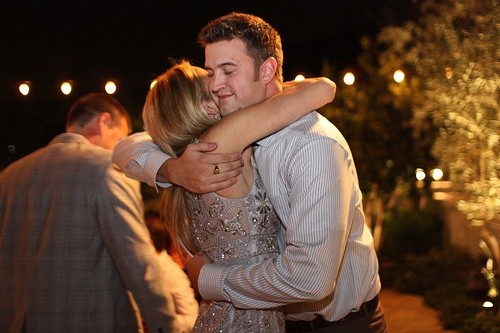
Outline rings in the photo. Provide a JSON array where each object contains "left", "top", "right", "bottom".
[{"left": 214, "top": 165, "right": 221, "bottom": 174}]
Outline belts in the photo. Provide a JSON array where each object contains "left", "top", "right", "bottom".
[{"left": 285, "top": 295, "right": 378, "bottom": 333}]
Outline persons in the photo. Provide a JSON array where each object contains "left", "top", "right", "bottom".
[
  {"left": 0, "top": 62, "right": 337, "bottom": 333},
  {"left": 112, "top": 12, "right": 388, "bottom": 333}
]
